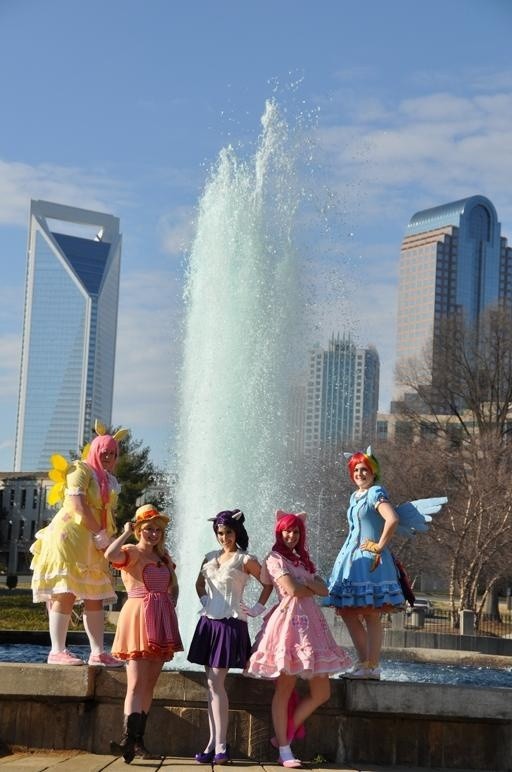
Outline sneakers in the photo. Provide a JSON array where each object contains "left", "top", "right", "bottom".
[
  {"left": 87, "top": 651, "right": 125, "bottom": 667},
  {"left": 339, "top": 663, "right": 381, "bottom": 680},
  {"left": 270, "top": 736, "right": 301, "bottom": 767},
  {"left": 48, "top": 648, "right": 83, "bottom": 665}
]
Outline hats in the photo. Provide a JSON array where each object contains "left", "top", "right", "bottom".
[{"left": 131, "top": 504, "right": 170, "bottom": 529}]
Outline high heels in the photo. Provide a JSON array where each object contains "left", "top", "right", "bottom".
[
  {"left": 195, "top": 746, "right": 214, "bottom": 763},
  {"left": 213, "top": 741, "right": 232, "bottom": 765}
]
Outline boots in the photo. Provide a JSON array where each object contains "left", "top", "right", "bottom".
[
  {"left": 109, "top": 711, "right": 141, "bottom": 764},
  {"left": 135, "top": 710, "right": 161, "bottom": 759}
]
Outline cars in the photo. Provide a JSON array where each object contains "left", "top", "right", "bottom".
[{"left": 406, "top": 598, "right": 436, "bottom": 618}]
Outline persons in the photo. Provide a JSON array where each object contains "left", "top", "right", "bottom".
[
  {"left": 245, "top": 515, "right": 353, "bottom": 768},
  {"left": 29, "top": 434, "right": 126, "bottom": 669},
  {"left": 104, "top": 502, "right": 184, "bottom": 762},
  {"left": 322, "top": 453, "right": 406, "bottom": 678},
  {"left": 187, "top": 509, "right": 273, "bottom": 762}
]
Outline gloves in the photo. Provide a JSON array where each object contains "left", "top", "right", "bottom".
[
  {"left": 197, "top": 596, "right": 210, "bottom": 615},
  {"left": 94, "top": 529, "right": 110, "bottom": 551},
  {"left": 240, "top": 600, "right": 266, "bottom": 617}
]
